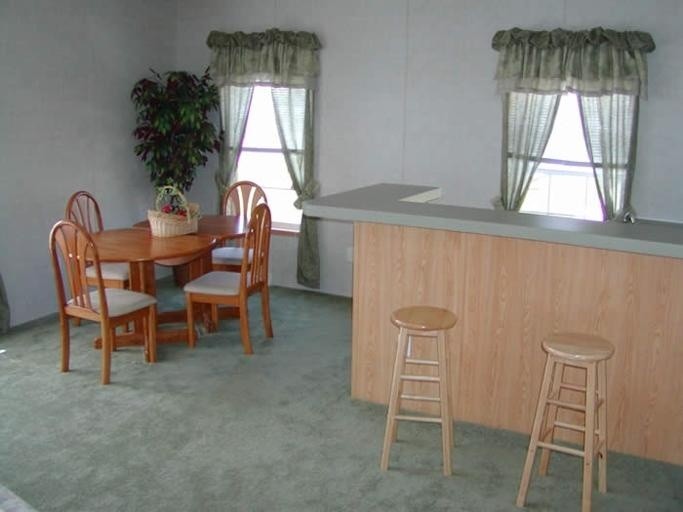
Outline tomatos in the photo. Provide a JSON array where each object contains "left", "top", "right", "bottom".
[{"left": 162, "top": 204, "right": 174, "bottom": 214}]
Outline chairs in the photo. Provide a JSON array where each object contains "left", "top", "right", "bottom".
[
  {"left": 181, "top": 203, "right": 274, "bottom": 356},
  {"left": 62, "top": 189, "right": 134, "bottom": 337},
  {"left": 48, "top": 218, "right": 159, "bottom": 387},
  {"left": 210, "top": 179, "right": 268, "bottom": 273}
]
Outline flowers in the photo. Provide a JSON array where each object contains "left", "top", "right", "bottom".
[
  {"left": 160, "top": 202, "right": 188, "bottom": 218},
  {"left": 128, "top": 61, "right": 225, "bottom": 287}
]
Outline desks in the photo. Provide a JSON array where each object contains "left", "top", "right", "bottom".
[{"left": 69, "top": 207, "right": 271, "bottom": 348}]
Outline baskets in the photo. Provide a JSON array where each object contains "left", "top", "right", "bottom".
[{"left": 147, "top": 186, "right": 200, "bottom": 238}]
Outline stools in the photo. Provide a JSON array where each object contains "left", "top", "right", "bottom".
[
  {"left": 375, "top": 304, "right": 460, "bottom": 479},
  {"left": 512, "top": 329, "right": 614, "bottom": 508}
]
